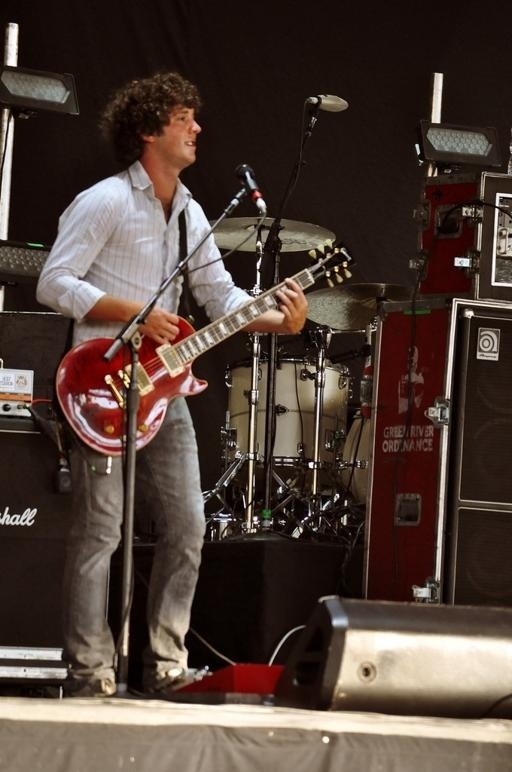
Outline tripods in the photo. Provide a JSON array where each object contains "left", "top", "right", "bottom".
[{"left": 200, "top": 229, "right": 366, "bottom": 543}]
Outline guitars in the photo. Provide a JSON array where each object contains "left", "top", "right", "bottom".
[{"left": 54, "top": 237, "right": 354, "bottom": 457}]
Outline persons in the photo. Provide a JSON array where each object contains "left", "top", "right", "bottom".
[{"left": 35, "top": 69, "right": 308, "bottom": 698}]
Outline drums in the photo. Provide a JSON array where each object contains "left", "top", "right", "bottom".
[
  {"left": 217, "top": 356, "right": 348, "bottom": 468},
  {"left": 335, "top": 412, "right": 374, "bottom": 506}
]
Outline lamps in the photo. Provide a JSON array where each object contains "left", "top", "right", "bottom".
[
  {"left": 0, "top": 66, "right": 80, "bottom": 129},
  {"left": 415, "top": 119, "right": 502, "bottom": 168}
]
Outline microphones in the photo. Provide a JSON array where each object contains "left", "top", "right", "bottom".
[
  {"left": 306, "top": 94, "right": 349, "bottom": 112},
  {"left": 236, "top": 163, "right": 267, "bottom": 212}
]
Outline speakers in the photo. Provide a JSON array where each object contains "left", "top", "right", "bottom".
[{"left": 361, "top": 298, "right": 512, "bottom": 607}]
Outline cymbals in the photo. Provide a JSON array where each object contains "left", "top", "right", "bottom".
[
  {"left": 206, "top": 217, "right": 338, "bottom": 254},
  {"left": 303, "top": 280, "right": 416, "bottom": 331}
]
